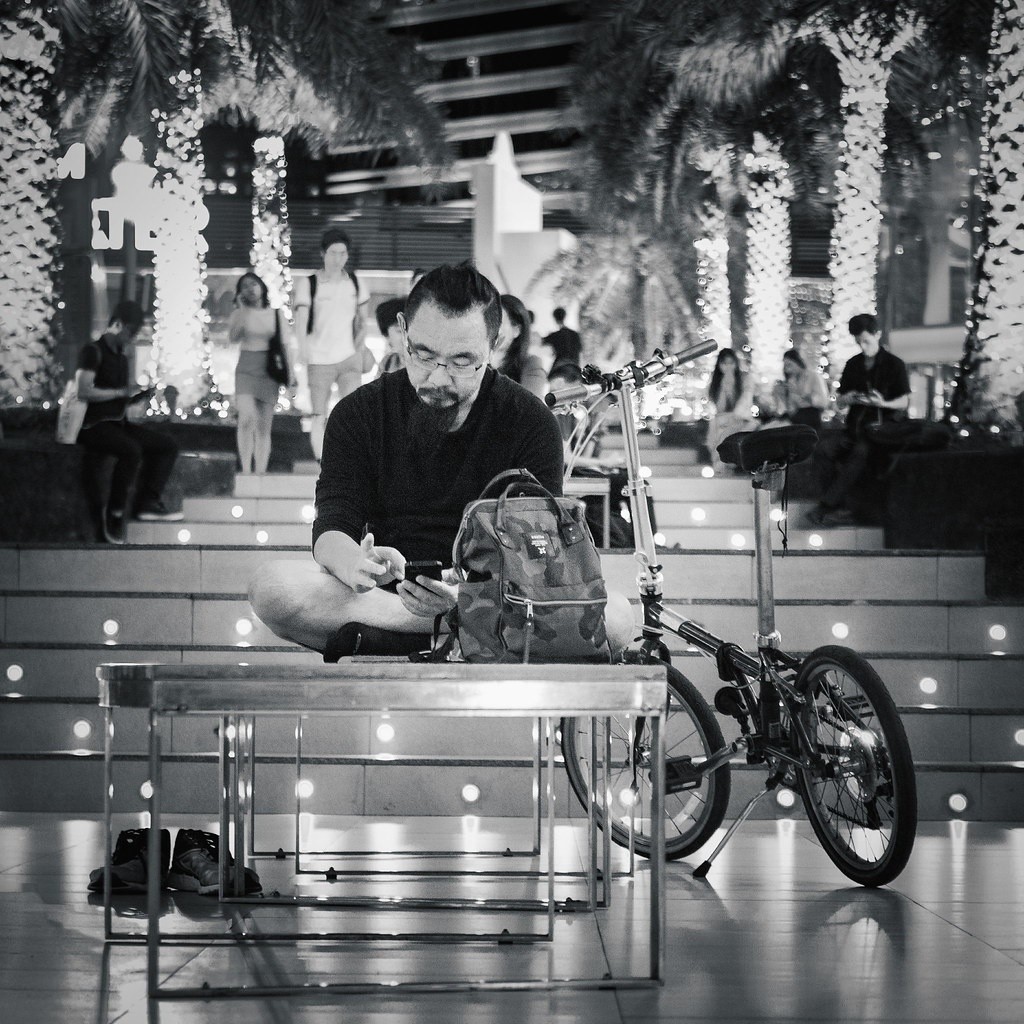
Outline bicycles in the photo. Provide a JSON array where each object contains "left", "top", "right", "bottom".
[{"left": 545, "top": 340, "right": 917, "bottom": 889}]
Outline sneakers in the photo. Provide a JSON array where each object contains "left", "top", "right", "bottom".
[
  {"left": 86, "top": 828, "right": 171, "bottom": 894},
  {"left": 168, "top": 828, "right": 262, "bottom": 896}
]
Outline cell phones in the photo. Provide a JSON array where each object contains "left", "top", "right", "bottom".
[{"left": 404, "top": 561, "right": 445, "bottom": 595}]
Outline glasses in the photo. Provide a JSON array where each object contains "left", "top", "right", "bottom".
[{"left": 404, "top": 327, "right": 489, "bottom": 378}]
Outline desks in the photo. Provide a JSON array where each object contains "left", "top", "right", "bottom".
[
  {"left": 564, "top": 476, "right": 610, "bottom": 549},
  {"left": 95, "top": 663, "right": 668, "bottom": 998}
]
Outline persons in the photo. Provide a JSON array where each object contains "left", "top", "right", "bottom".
[
  {"left": 228, "top": 272, "right": 296, "bottom": 475},
  {"left": 770, "top": 350, "right": 828, "bottom": 429},
  {"left": 296, "top": 228, "right": 372, "bottom": 466},
  {"left": 77, "top": 302, "right": 184, "bottom": 543},
  {"left": 246, "top": 257, "right": 664, "bottom": 656},
  {"left": 803, "top": 313, "right": 911, "bottom": 527},
  {"left": 706, "top": 349, "right": 760, "bottom": 470}
]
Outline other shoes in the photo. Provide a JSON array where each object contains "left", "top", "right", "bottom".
[
  {"left": 103, "top": 506, "right": 123, "bottom": 543},
  {"left": 804, "top": 508, "right": 830, "bottom": 524},
  {"left": 137, "top": 501, "right": 186, "bottom": 522}
]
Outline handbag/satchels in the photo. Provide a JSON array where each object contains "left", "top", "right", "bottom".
[
  {"left": 454, "top": 467, "right": 618, "bottom": 663},
  {"left": 56, "top": 345, "right": 101, "bottom": 443},
  {"left": 267, "top": 308, "right": 291, "bottom": 384}
]
